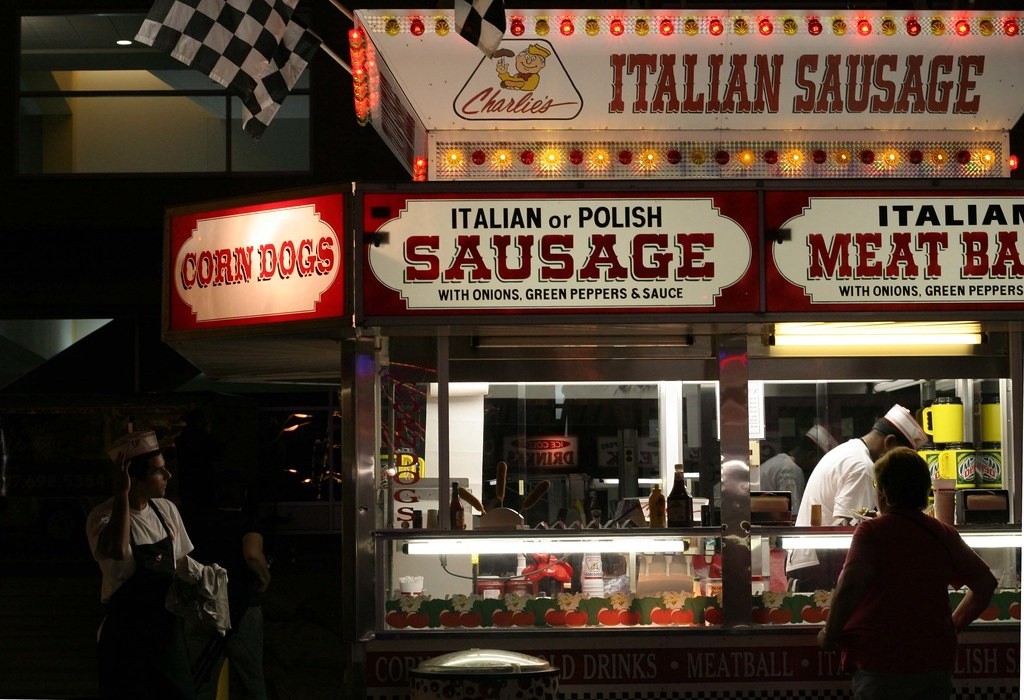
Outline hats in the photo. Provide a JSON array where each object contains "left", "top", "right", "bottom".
[
  {"left": 804, "top": 424, "right": 838, "bottom": 456},
  {"left": 107, "top": 431, "right": 158, "bottom": 464},
  {"left": 873, "top": 403, "right": 928, "bottom": 450}
]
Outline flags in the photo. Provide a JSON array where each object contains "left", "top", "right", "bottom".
[
  {"left": 454, "top": 1, "right": 508, "bottom": 59},
  {"left": 136, "top": 0, "right": 324, "bottom": 140}
]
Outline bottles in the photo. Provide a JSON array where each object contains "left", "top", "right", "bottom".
[
  {"left": 503, "top": 573, "right": 534, "bottom": 596},
  {"left": 919, "top": 441, "right": 975, "bottom": 489},
  {"left": 668, "top": 463, "right": 693, "bottom": 527},
  {"left": 475, "top": 575, "right": 504, "bottom": 599},
  {"left": 650, "top": 483, "right": 665, "bottom": 528},
  {"left": 449, "top": 481, "right": 463, "bottom": 532},
  {"left": 976, "top": 444, "right": 1002, "bottom": 488},
  {"left": 979, "top": 392, "right": 1001, "bottom": 441}
]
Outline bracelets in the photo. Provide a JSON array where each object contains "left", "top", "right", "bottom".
[{"left": 823, "top": 629, "right": 832, "bottom": 638}]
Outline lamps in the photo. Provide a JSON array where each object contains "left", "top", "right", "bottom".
[
  {"left": 469, "top": 336, "right": 697, "bottom": 349},
  {"left": 767, "top": 333, "right": 990, "bottom": 346}
]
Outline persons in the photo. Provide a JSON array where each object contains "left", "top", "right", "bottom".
[
  {"left": 793, "top": 403, "right": 929, "bottom": 528},
  {"left": 86, "top": 429, "right": 229, "bottom": 700},
  {"left": 760, "top": 424, "right": 839, "bottom": 523},
  {"left": 225, "top": 476, "right": 287, "bottom": 700},
  {"left": 815, "top": 447, "right": 998, "bottom": 700}
]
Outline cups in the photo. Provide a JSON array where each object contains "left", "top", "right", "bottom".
[
  {"left": 933, "top": 489, "right": 955, "bottom": 526},
  {"left": 921, "top": 396, "right": 965, "bottom": 444},
  {"left": 411, "top": 510, "right": 422, "bottom": 528},
  {"left": 427, "top": 509, "right": 438, "bottom": 528},
  {"left": 812, "top": 504, "right": 822, "bottom": 526}
]
